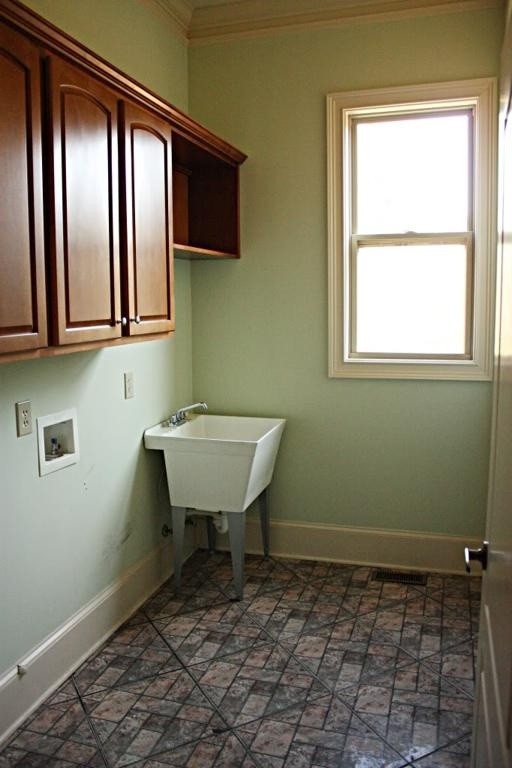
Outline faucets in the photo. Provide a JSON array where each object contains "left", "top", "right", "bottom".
[{"left": 176, "top": 401, "right": 209, "bottom": 420}]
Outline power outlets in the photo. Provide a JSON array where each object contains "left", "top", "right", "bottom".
[
  {"left": 15, "top": 399, "right": 32, "bottom": 436},
  {"left": 124, "top": 371, "right": 135, "bottom": 398}
]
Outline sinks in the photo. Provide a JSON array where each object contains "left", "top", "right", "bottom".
[{"left": 143, "top": 412, "right": 288, "bottom": 513}]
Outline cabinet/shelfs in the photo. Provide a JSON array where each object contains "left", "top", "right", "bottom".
[
  {"left": 0, "top": 21, "right": 49, "bottom": 353},
  {"left": 171, "top": 128, "right": 239, "bottom": 257},
  {"left": 48, "top": 59, "right": 173, "bottom": 346}
]
